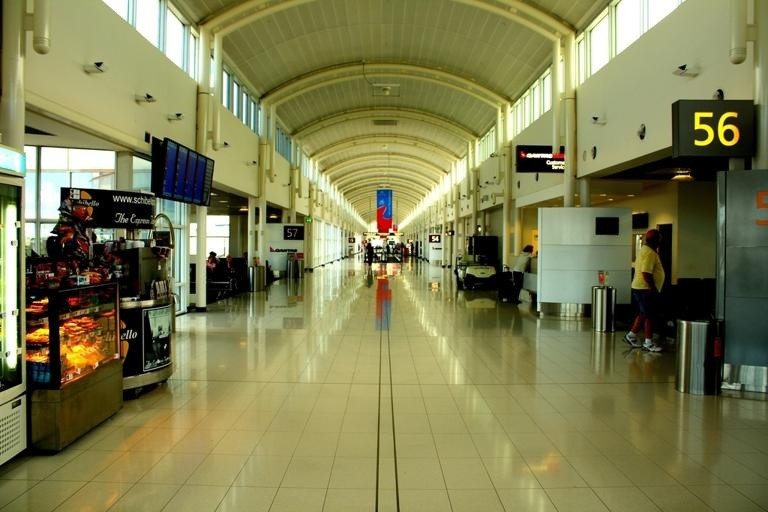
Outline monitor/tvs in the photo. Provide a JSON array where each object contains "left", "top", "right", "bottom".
[{"left": 632, "top": 213, "right": 648, "bottom": 229}]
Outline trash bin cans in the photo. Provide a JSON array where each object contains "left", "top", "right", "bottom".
[
  {"left": 592, "top": 286, "right": 617, "bottom": 332},
  {"left": 250, "top": 266, "right": 264, "bottom": 292},
  {"left": 675, "top": 320, "right": 725, "bottom": 395},
  {"left": 286, "top": 260, "right": 304, "bottom": 278}
]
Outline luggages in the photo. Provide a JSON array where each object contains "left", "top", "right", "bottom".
[{"left": 497, "top": 266, "right": 512, "bottom": 302}]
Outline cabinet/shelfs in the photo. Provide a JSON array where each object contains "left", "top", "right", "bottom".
[{"left": 26, "top": 283, "right": 123, "bottom": 457}]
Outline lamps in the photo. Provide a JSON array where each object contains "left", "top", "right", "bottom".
[
  {"left": 248, "top": 161, "right": 256, "bottom": 166},
  {"left": 674, "top": 62, "right": 699, "bottom": 74},
  {"left": 592, "top": 116, "right": 606, "bottom": 123},
  {"left": 363, "top": 61, "right": 400, "bottom": 97},
  {"left": 136, "top": 93, "right": 156, "bottom": 102},
  {"left": 220, "top": 141, "right": 230, "bottom": 148},
  {"left": 85, "top": 62, "right": 107, "bottom": 72},
  {"left": 168, "top": 113, "right": 184, "bottom": 120}
]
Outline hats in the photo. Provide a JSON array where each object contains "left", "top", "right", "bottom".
[{"left": 646, "top": 229, "right": 663, "bottom": 240}]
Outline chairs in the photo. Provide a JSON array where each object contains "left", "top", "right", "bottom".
[{"left": 190, "top": 258, "right": 249, "bottom": 304}]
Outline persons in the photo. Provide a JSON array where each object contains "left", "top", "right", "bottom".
[
  {"left": 386, "top": 244, "right": 390, "bottom": 254},
  {"left": 510, "top": 245, "right": 538, "bottom": 304},
  {"left": 207, "top": 252, "right": 219, "bottom": 272},
  {"left": 367, "top": 243, "right": 374, "bottom": 266},
  {"left": 623, "top": 230, "right": 669, "bottom": 352},
  {"left": 223, "top": 256, "right": 235, "bottom": 276}
]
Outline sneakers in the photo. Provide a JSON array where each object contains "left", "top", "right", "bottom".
[{"left": 623, "top": 334, "right": 664, "bottom": 352}]
